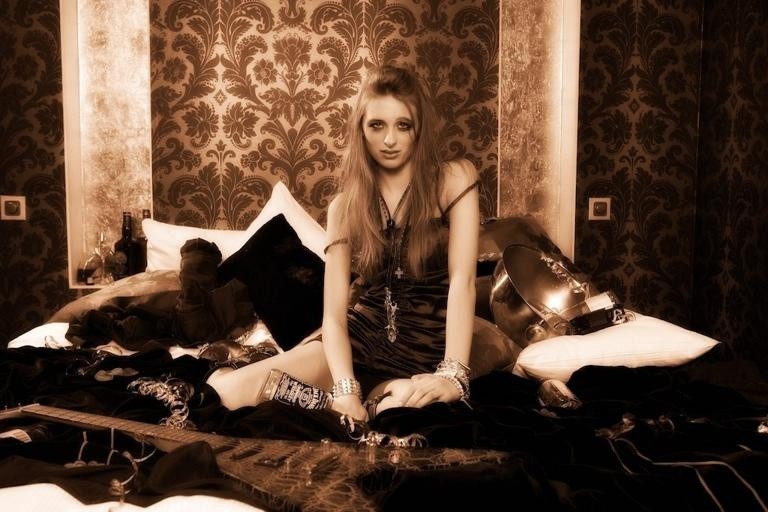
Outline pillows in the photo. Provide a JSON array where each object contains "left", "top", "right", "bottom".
[
  {"left": 518, "top": 316, "right": 719, "bottom": 384},
  {"left": 141, "top": 181, "right": 356, "bottom": 350}
]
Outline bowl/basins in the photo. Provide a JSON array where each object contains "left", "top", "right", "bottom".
[{"left": 488, "top": 242, "right": 592, "bottom": 349}]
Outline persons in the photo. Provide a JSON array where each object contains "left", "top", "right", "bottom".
[{"left": 194, "top": 65, "right": 482, "bottom": 423}]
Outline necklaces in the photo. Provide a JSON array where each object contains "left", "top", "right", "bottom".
[{"left": 372, "top": 180, "right": 415, "bottom": 342}]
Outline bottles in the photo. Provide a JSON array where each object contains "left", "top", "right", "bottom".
[
  {"left": 114, "top": 207, "right": 153, "bottom": 280},
  {"left": 77, "top": 231, "right": 115, "bottom": 285},
  {"left": 535, "top": 376, "right": 584, "bottom": 411},
  {"left": 256, "top": 368, "right": 334, "bottom": 410}
]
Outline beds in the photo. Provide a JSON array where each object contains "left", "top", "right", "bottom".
[{"left": 0, "top": 270, "right": 768, "bottom": 512}]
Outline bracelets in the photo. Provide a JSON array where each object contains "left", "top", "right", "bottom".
[
  {"left": 433, "top": 356, "right": 473, "bottom": 399},
  {"left": 331, "top": 378, "right": 364, "bottom": 401}
]
